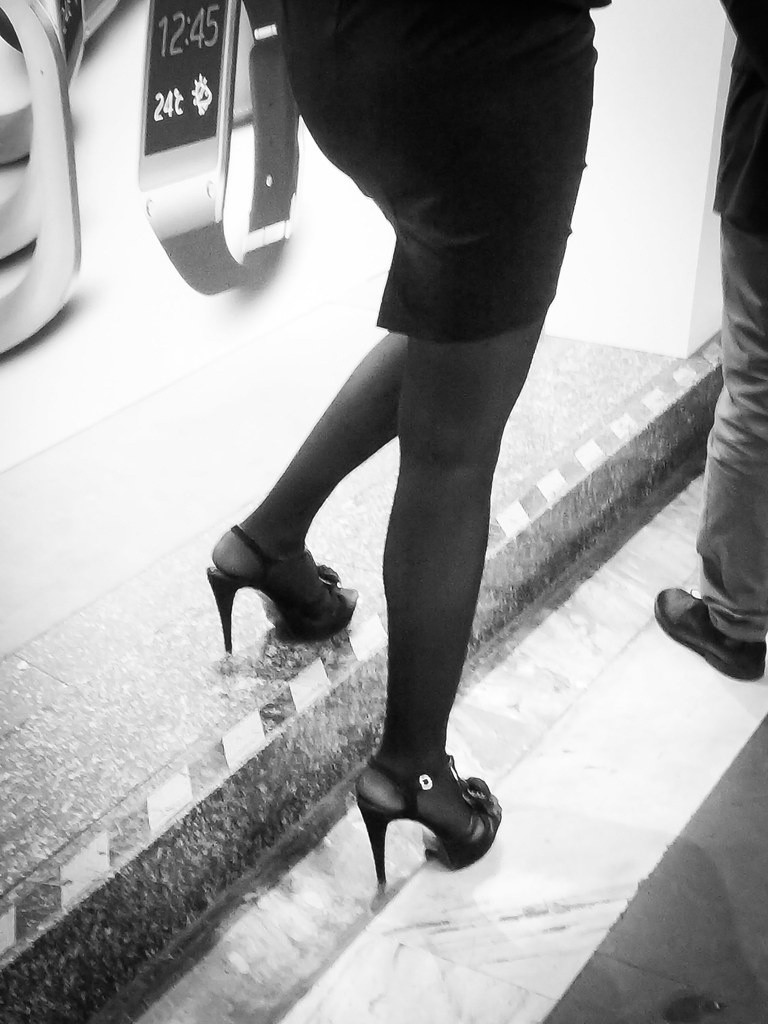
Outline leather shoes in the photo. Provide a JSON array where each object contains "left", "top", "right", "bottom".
[{"left": 655, "top": 588, "right": 768, "bottom": 681}]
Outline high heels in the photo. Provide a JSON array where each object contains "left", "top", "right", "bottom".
[
  {"left": 356, "top": 753, "right": 503, "bottom": 883},
  {"left": 206, "top": 525, "right": 358, "bottom": 654}
]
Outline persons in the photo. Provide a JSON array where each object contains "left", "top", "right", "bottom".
[
  {"left": 204, "top": 0, "right": 613, "bottom": 886},
  {"left": 655, "top": 0, "right": 768, "bottom": 681}
]
